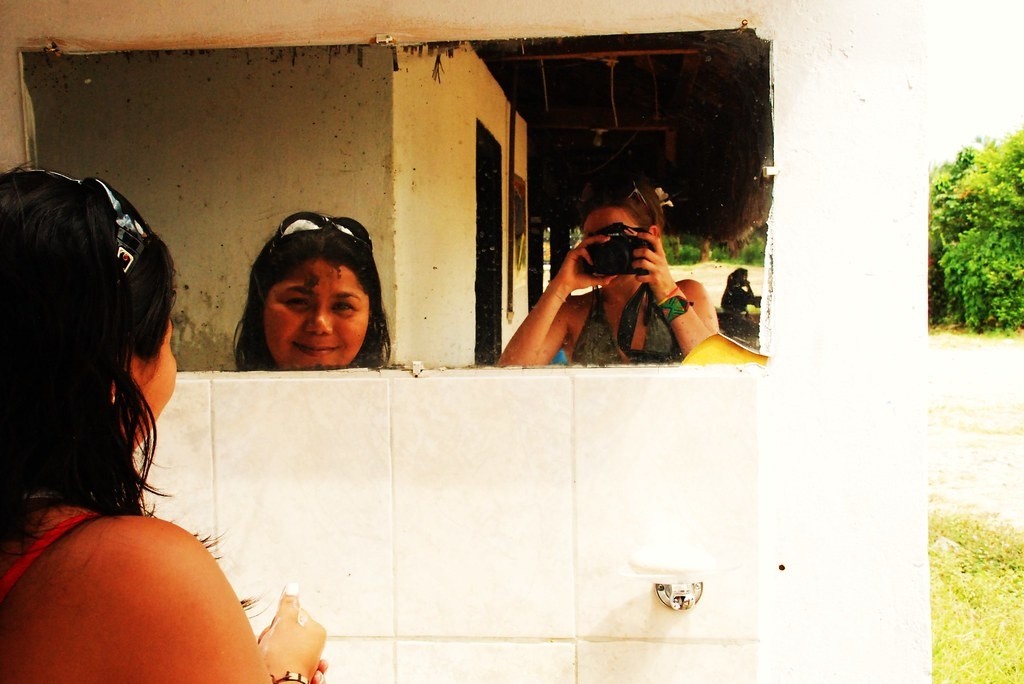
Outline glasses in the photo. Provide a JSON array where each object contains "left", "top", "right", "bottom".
[
  {"left": 23, "top": 167, "right": 151, "bottom": 287},
  {"left": 589, "top": 180, "right": 650, "bottom": 204},
  {"left": 262, "top": 211, "right": 373, "bottom": 263}
]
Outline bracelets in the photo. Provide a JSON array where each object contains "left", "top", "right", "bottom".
[
  {"left": 546, "top": 286, "right": 566, "bottom": 302},
  {"left": 657, "top": 286, "right": 694, "bottom": 324},
  {"left": 271, "top": 671, "right": 309, "bottom": 684}
]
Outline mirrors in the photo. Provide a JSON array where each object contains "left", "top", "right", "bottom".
[{"left": 16, "top": 23, "right": 776, "bottom": 373}]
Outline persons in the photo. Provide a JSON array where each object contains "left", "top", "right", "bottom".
[
  {"left": 721, "top": 268, "right": 762, "bottom": 333},
  {"left": 0, "top": 168, "right": 329, "bottom": 684},
  {"left": 231, "top": 211, "right": 392, "bottom": 371},
  {"left": 496, "top": 179, "right": 720, "bottom": 368}
]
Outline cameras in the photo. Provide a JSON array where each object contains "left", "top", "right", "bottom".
[{"left": 583, "top": 221, "right": 656, "bottom": 276}]
